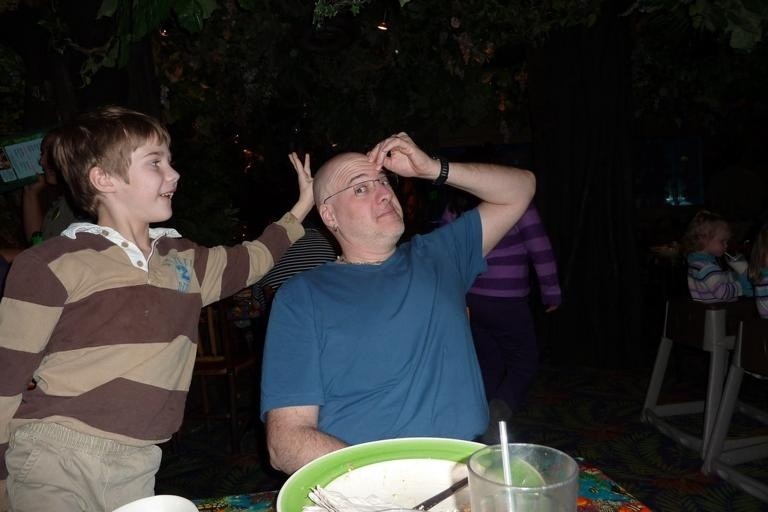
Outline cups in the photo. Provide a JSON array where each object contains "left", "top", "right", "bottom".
[
  {"left": 727, "top": 254, "right": 749, "bottom": 274},
  {"left": 467, "top": 441, "right": 579, "bottom": 512}
]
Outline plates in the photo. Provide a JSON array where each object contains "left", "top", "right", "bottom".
[{"left": 274, "top": 436, "right": 547, "bottom": 512}]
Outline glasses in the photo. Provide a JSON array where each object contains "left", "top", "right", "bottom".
[{"left": 322, "top": 170, "right": 399, "bottom": 206}]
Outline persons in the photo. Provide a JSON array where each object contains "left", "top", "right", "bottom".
[
  {"left": 256, "top": 129, "right": 536, "bottom": 476},
  {"left": 0, "top": 135, "right": 768, "bottom": 433},
  {"left": 0, "top": 104, "right": 315, "bottom": 512}
]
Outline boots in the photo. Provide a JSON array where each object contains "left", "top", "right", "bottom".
[{"left": 479, "top": 396, "right": 517, "bottom": 444}]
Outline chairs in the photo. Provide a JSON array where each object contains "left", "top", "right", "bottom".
[
  {"left": 639, "top": 288, "right": 768, "bottom": 501},
  {"left": 193, "top": 297, "right": 260, "bottom": 454}
]
